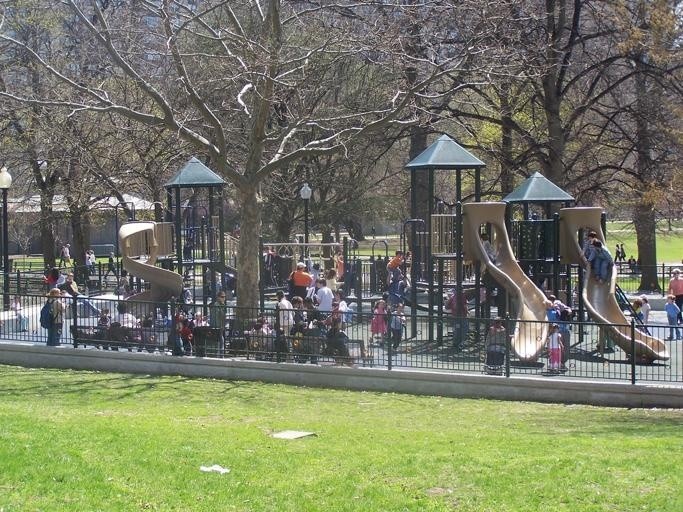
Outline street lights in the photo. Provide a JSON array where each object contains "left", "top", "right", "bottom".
[
  {"left": 0, "top": 166, "right": 12, "bottom": 309},
  {"left": 299, "top": 183, "right": 312, "bottom": 269}
]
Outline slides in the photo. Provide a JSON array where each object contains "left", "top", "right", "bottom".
[
  {"left": 118, "top": 222, "right": 183, "bottom": 327},
  {"left": 559, "top": 207, "right": 672, "bottom": 361},
  {"left": 463, "top": 201, "right": 554, "bottom": 362}
]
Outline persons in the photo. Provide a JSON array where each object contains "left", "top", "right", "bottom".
[
  {"left": 485, "top": 316, "right": 508, "bottom": 366},
  {"left": 445, "top": 284, "right": 469, "bottom": 352},
  {"left": 664, "top": 269, "right": 683, "bottom": 341},
  {"left": 481, "top": 232, "right": 497, "bottom": 263},
  {"left": 582, "top": 231, "right": 613, "bottom": 285},
  {"left": 632, "top": 294, "right": 651, "bottom": 334},
  {"left": 42, "top": 242, "right": 358, "bottom": 371},
  {"left": 546, "top": 294, "right": 574, "bottom": 370},
  {"left": 12, "top": 294, "right": 28, "bottom": 331},
  {"left": 614, "top": 243, "right": 636, "bottom": 273},
  {"left": 369, "top": 250, "right": 410, "bottom": 354}
]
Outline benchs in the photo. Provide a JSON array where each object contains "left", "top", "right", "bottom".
[
  {"left": 243, "top": 332, "right": 375, "bottom": 368},
  {"left": 69, "top": 325, "right": 170, "bottom": 354}
]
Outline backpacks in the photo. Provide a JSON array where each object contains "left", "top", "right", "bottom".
[{"left": 40, "top": 300, "right": 59, "bottom": 330}]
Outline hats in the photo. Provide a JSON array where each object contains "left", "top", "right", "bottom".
[{"left": 297, "top": 262, "right": 306, "bottom": 268}]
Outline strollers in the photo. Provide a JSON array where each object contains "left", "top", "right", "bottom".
[{"left": 483, "top": 343, "right": 506, "bottom": 375}]
